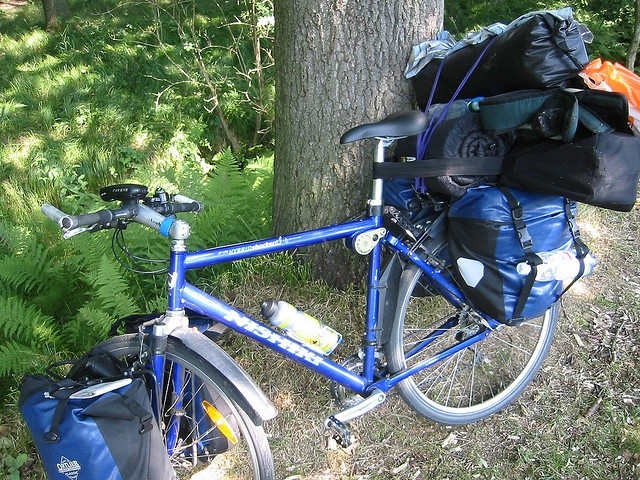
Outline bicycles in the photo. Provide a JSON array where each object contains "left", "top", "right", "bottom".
[{"left": 41, "top": 108, "right": 559, "bottom": 480}]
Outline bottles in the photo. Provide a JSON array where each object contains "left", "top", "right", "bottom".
[
  {"left": 259, "top": 300, "right": 341, "bottom": 355},
  {"left": 517, "top": 249, "right": 594, "bottom": 282}
]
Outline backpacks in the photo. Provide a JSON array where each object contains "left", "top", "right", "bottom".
[
  {"left": 17, "top": 345, "right": 178, "bottom": 480},
  {"left": 448, "top": 184, "right": 597, "bottom": 326}
]
